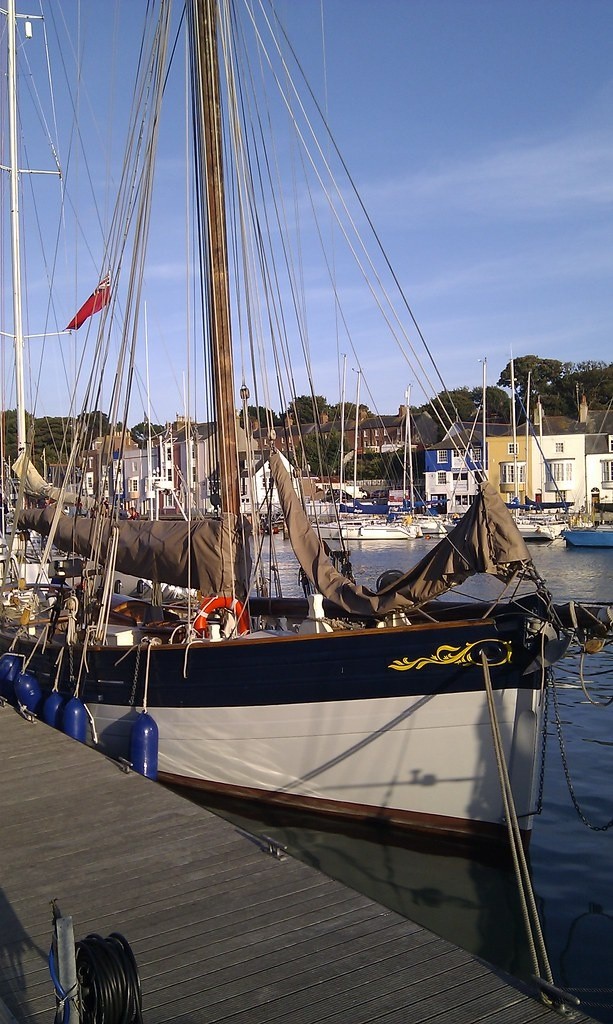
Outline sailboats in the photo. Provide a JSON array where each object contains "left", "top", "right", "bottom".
[{"left": 0, "top": 0, "right": 612, "bottom": 849}]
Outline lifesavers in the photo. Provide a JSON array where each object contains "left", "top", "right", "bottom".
[{"left": 193, "top": 596, "right": 251, "bottom": 641}]
